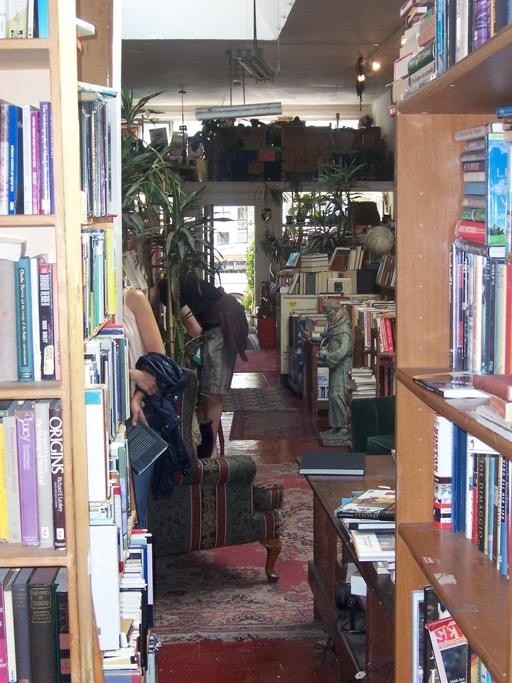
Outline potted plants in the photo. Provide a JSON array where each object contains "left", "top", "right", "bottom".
[
  {"left": 120, "top": 87, "right": 235, "bottom": 370},
  {"left": 257, "top": 156, "right": 364, "bottom": 269}
]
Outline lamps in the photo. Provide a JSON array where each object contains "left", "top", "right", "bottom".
[{"left": 355, "top": 56, "right": 367, "bottom": 111}]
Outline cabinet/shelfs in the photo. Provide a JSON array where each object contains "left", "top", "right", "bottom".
[
  {"left": 278, "top": 249, "right": 398, "bottom": 413},
  {"left": 388, "top": 5, "right": 512, "bottom": 638},
  {"left": 4, "top": 0, "right": 159, "bottom": 639}
]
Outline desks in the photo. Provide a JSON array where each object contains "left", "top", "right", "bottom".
[{"left": 291, "top": 450, "right": 392, "bottom": 683}]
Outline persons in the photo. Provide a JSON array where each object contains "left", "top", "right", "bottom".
[{"left": 149, "top": 262, "right": 249, "bottom": 461}]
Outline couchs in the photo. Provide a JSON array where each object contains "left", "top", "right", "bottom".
[{"left": 133, "top": 363, "right": 284, "bottom": 585}]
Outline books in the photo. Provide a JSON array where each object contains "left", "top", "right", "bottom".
[
  {"left": 270, "top": 1, "right": 511, "bottom": 681},
  {"left": 0, "top": 0, "right": 169, "bottom": 683}
]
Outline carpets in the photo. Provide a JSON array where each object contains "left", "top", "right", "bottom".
[
  {"left": 220, "top": 387, "right": 318, "bottom": 441},
  {"left": 148, "top": 461, "right": 339, "bottom": 640},
  {"left": 235, "top": 348, "right": 280, "bottom": 373}
]
